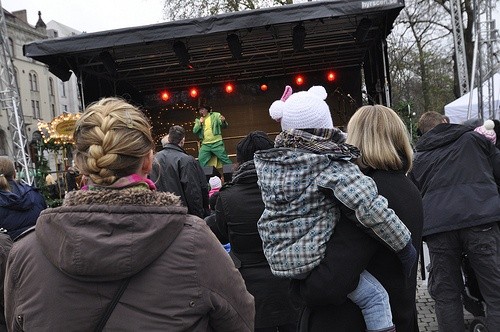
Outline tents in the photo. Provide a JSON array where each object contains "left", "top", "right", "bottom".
[{"left": 444, "top": 71, "right": 500, "bottom": 126}]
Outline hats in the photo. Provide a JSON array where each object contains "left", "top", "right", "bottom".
[
  {"left": 474, "top": 119, "right": 496, "bottom": 144},
  {"left": 237, "top": 131, "right": 276, "bottom": 163},
  {"left": 195, "top": 104, "right": 210, "bottom": 113},
  {"left": 269, "top": 85, "right": 333, "bottom": 130},
  {"left": 209, "top": 175, "right": 222, "bottom": 190}
]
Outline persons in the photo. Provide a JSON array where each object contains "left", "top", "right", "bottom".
[
  {"left": 216, "top": 129, "right": 297, "bottom": 331},
  {"left": 464, "top": 115, "right": 500, "bottom": 149},
  {"left": 0, "top": 155, "right": 47, "bottom": 239},
  {"left": 66, "top": 166, "right": 78, "bottom": 192},
  {"left": 193, "top": 104, "right": 234, "bottom": 168},
  {"left": 2, "top": 97, "right": 256, "bottom": 332},
  {"left": 252, "top": 86, "right": 419, "bottom": 332},
  {"left": 45, "top": 168, "right": 56, "bottom": 187},
  {"left": 161, "top": 133, "right": 232, "bottom": 256},
  {"left": 146, "top": 126, "right": 205, "bottom": 219},
  {"left": 410, "top": 111, "right": 500, "bottom": 331},
  {"left": 286, "top": 104, "right": 424, "bottom": 331}
]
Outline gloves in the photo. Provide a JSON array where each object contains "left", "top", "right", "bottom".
[{"left": 397, "top": 237, "right": 417, "bottom": 276}]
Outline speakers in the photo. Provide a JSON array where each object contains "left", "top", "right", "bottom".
[
  {"left": 223, "top": 164, "right": 237, "bottom": 183},
  {"left": 202, "top": 166, "right": 221, "bottom": 184}
]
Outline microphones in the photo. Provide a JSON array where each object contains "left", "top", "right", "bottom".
[
  {"left": 330, "top": 87, "right": 339, "bottom": 96},
  {"left": 201, "top": 113, "right": 204, "bottom": 126}
]
